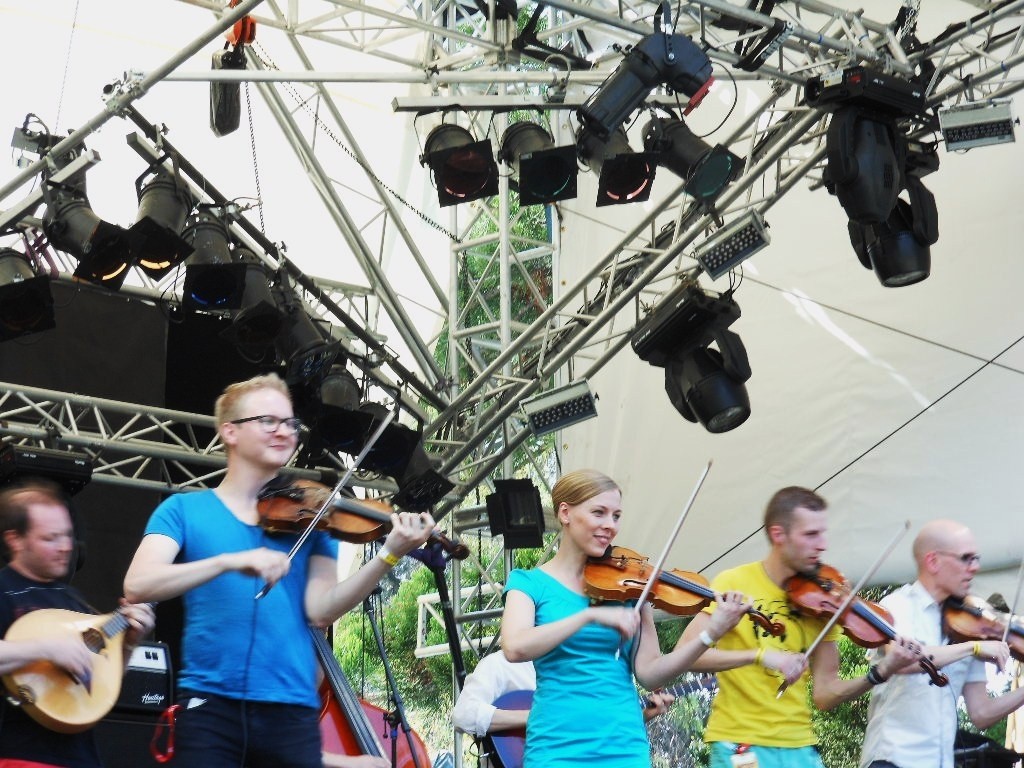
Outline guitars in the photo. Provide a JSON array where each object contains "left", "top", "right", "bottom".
[
  {"left": 482, "top": 678, "right": 719, "bottom": 767},
  {"left": 3, "top": 601, "right": 160, "bottom": 734}
]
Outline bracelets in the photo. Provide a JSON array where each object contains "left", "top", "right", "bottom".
[
  {"left": 378, "top": 548, "right": 401, "bottom": 566},
  {"left": 868, "top": 663, "right": 888, "bottom": 684},
  {"left": 700, "top": 631, "right": 717, "bottom": 649},
  {"left": 753, "top": 648, "right": 766, "bottom": 667},
  {"left": 970, "top": 641, "right": 980, "bottom": 658}
]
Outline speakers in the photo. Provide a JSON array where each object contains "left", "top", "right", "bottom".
[
  {"left": 93, "top": 709, "right": 174, "bottom": 768},
  {"left": 488, "top": 478, "right": 546, "bottom": 549}
]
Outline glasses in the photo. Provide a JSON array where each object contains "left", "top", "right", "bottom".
[
  {"left": 231, "top": 415, "right": 301, "bottom": 432},
  {"left": 936, "top": 550, "right": 981, "bottom": 565}
]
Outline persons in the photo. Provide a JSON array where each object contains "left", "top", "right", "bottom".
[
  {"left": 857, "top": 518, "right": 1024, "bottom": 768},
  {"left": 320, "top": 750, "right": 392, "bottom": 768},
  {"left": 124, "top": 374, "right": 436, "bottom": 768},
  {"left": 0, "top": 484, "right": 156, "bottom": 767},
  {"left": 452, "top": 652, "right": 676, "bottom": 768},
  {"left": 669, "top": 485, "right": 924, "bottom": 768},
  {"left": 500, "top": 468, "right": 753, "bottom": 768}
]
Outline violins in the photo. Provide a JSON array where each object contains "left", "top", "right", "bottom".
[
  {"left": 787, "top": 563, "right": 948, "bottom": 687},
  {"left": 256, "top": 473, "right": 470, "bottom": 560},
  {"left": 942, "top": 594, "right": 1024, "bottom": 662},
  {"left": 584, "top": 544, "right": 785, "bottom": 638}
]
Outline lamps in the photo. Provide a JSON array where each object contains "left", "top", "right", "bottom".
[{"left": 9, "top": 0, "right": 940, "bottom": 548}]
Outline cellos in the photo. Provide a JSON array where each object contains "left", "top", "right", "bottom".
[{"left": 308, "top": 626, "right": 430, "bottom": 768}]
